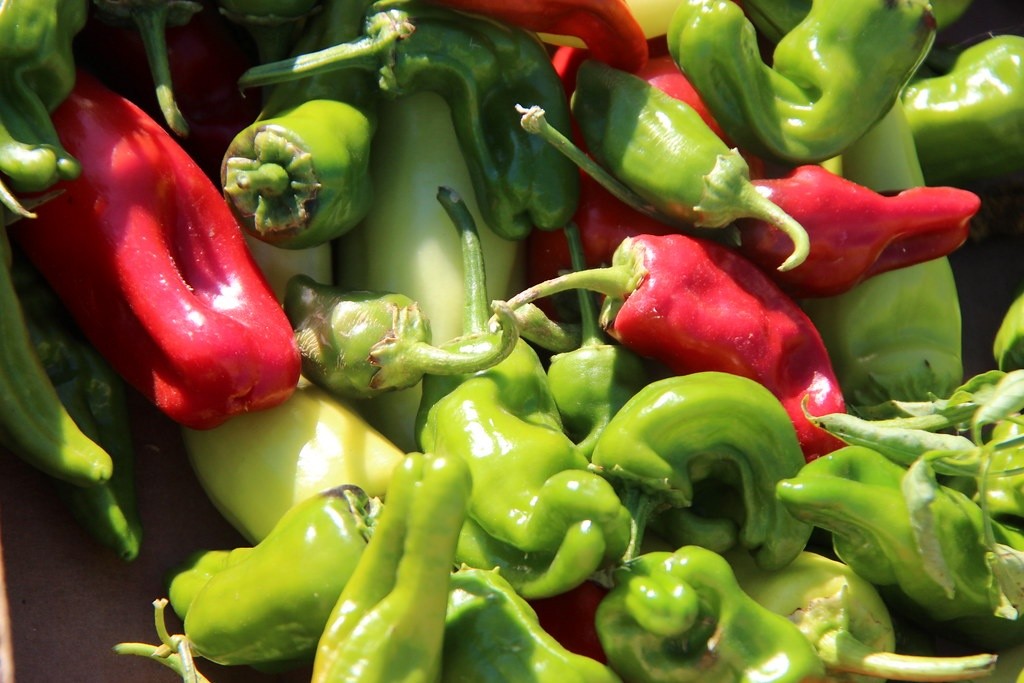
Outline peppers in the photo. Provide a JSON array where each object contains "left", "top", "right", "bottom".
[{"left": 0, "top": 0, "right": 1024, "bottom": 683}]
